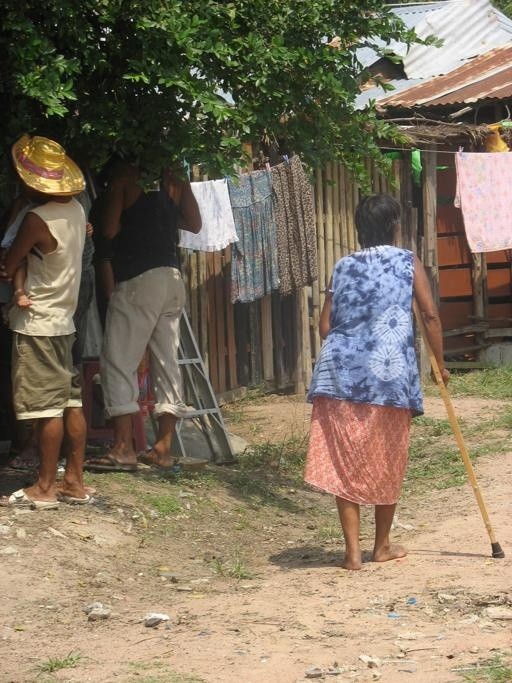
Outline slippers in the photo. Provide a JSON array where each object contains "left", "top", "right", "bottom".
[
  {"left": 57, "top": 493, "right": 90, "bottom": 506},
  {"left": 0, "top": 487, "right": 60, "bottom": 510},
  {"left": 83, "top": 450, "right": 179, "bottom": 472}
]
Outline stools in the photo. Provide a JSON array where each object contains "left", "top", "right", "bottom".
[{"left": 83, "top": 356, "right": 147, "bottom": 453}]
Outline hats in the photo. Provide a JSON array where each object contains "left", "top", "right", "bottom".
[{"left": 10, "top": 134, "right": 87, "bottom": 198}]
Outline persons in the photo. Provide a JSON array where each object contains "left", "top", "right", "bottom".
[
  {"left": 301, "top": 191, "right": 451, "bottom": 571},
  {"left": 1, "top": 121, "right": 202, "bottom": 509}
]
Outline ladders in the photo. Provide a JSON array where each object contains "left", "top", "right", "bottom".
[{"left": 85, "top": 168, "right": 237, "bottom": 465}]
